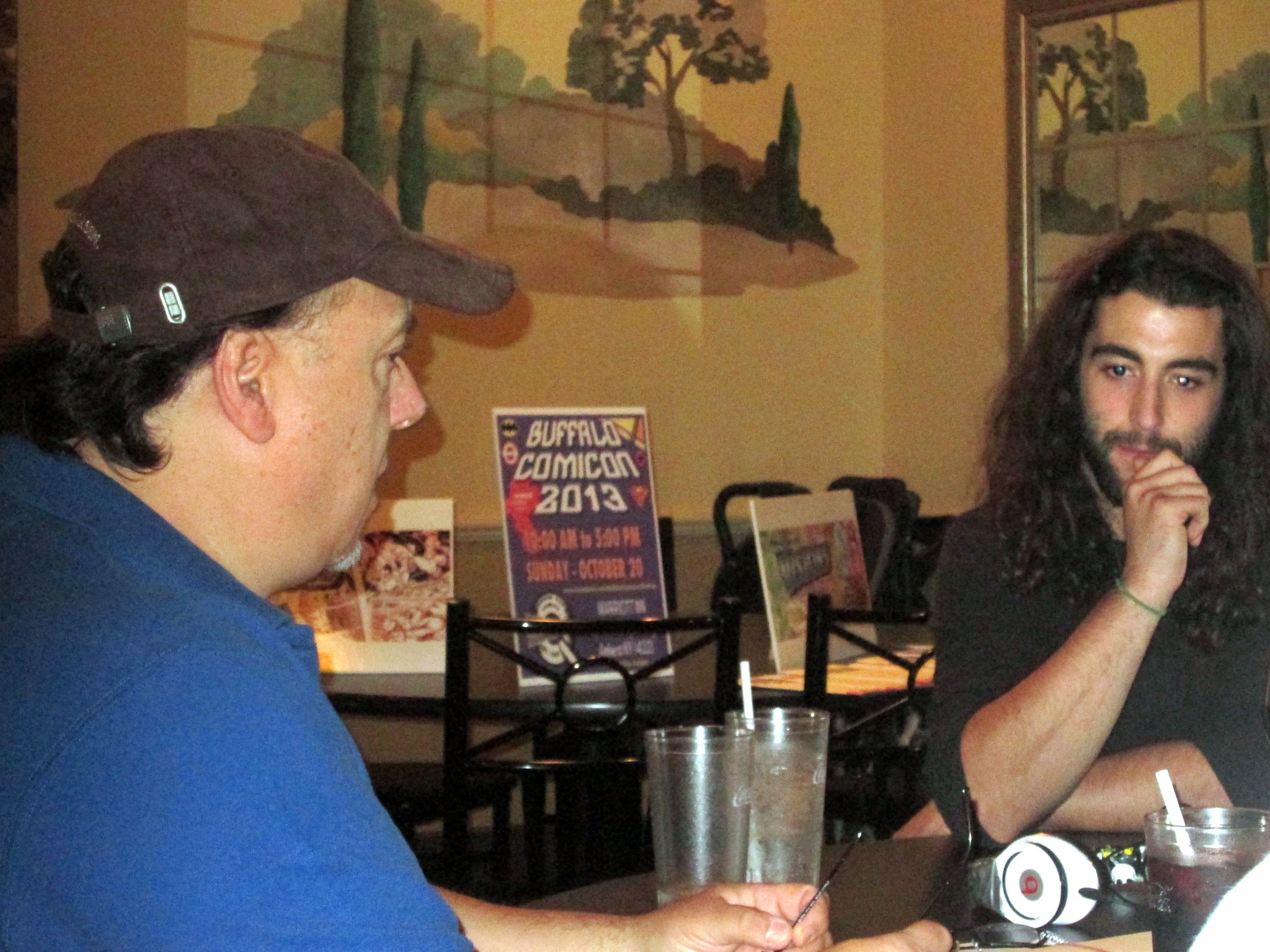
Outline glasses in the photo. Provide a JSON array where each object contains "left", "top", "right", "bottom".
[{"left": 948, "top": 923, "right": 1049, "bottom": 949}]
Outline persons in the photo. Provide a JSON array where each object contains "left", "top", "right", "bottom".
[
  {"left": 923, "top": 228, "right": 1270, "bottom": 855},
  {"left": 0, "top": 125, "right": 955, "bottom": 952}
]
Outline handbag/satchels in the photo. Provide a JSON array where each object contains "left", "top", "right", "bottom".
[{"left": 707, "top": 475, "right": 941, "bottom": 615}]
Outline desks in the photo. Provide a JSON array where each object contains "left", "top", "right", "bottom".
[
  {"left": 526, "top": 832, "right": 1148, "bottom": 952},
  {"left": 320, "top": 616, "right": 939, "bottom": 913}
]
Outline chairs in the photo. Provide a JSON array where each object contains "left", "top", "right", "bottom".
[{"left": 367, "top": 476, "right": 940, "bottom": 911}]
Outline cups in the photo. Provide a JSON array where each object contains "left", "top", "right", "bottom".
[
  {"left": 723, "top": 707, "right": 830, "bottom": 892},
  {"left": 642, "top": 725, "right": 755, "bottom": 909},
  {"left": 1143, "top": 807, "right": 1270, "bottom": 952}
]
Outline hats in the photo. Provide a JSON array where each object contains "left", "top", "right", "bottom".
[{"left": 46, "top": 128, "right": 517, "bottom": 347}]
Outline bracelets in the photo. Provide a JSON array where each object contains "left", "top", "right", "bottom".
[{"left": 1114, "top": 577, "right": 1168, "bottom": 616}]
